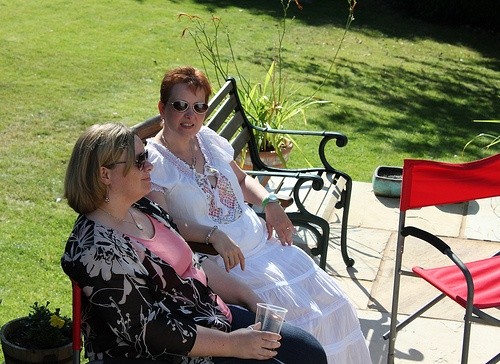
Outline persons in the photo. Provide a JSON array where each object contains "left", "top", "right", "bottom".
[
  {"left": 61, "top": 122, "right": 329, "bottom": 364},
  {"left": 142, "top": 66, "right": 372, "bottom": 364}
]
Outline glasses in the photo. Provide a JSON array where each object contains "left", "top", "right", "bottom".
[
  {"left": 163, "top": 100, "right": 209, "bottom": 114},
  {"left": 104, "top": 149, "right": 149, "bottom": 171}
]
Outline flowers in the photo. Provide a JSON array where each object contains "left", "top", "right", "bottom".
[{"left": 22, "top": 301, "right": 72, "bottom": 346}]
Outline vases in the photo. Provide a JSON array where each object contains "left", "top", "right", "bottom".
[{"left": 0, "top": 316, "right": 82, "bottom": 364}]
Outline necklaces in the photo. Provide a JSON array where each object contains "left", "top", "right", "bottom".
[
  {"left": 98, "top": 209, "right": 143, "bottom": 231},
  {"left": 162, "top": 136, "right": 196, "bottom": 168}
]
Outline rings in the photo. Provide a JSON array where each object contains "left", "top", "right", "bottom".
[
  {"left": 248, "top": 326, "right": 254, "bottom": 330},
  {"left": 287, "top": 227, "right": 290, "bottom": 230}
]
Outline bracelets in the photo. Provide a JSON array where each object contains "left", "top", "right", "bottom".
[{"left": 206, "top": 225, "right": 218, "bottom": 244}]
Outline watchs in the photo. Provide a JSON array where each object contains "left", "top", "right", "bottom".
[{"left": 261, "top": 193, "right": 282, "bottom": 210}]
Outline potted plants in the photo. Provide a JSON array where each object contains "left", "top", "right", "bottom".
[{"left": 178, "top": 0, "right": 356, "bottom": 183}]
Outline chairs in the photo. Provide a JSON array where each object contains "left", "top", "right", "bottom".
[
  {"left": 201, "top": 76, "right": 354, "bottom": 270},
  {"left": 383, "top": 153, "right": 500, "bottom": 364}
]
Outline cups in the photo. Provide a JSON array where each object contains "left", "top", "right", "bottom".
[{"left": 254, "top": 303, "right": 288, "bottom": 358}]
